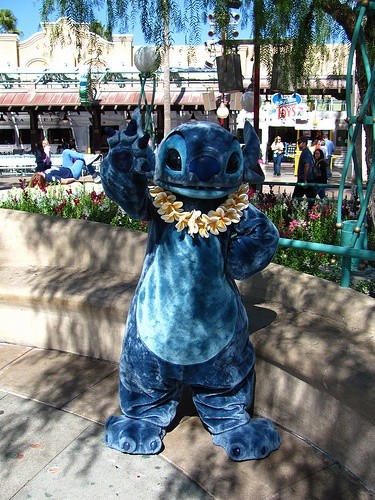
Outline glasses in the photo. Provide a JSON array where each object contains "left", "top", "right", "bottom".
[{"left": 298, "top": 141, "right": 305, "bottom": 144}]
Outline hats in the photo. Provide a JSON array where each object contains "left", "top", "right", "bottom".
[{"left": 297, "top": 136, "right": 308, "bottom": 142}]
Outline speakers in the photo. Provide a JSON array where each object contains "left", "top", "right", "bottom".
[{"left": 217, "top": 53, "right": 244, "bottom": 94}]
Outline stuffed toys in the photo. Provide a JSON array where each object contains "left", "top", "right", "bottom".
[{"left": 101, "top": 119, "right": 282, "bottom": 461}]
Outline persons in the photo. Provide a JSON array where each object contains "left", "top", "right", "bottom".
[{"left": 30, "top": 135, "right": 338, "bottom": 201}]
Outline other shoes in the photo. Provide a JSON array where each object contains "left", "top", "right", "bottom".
[
  {"left": 85, "top": 153, "right": 100, "bottom": 166},
  {"left": 93, "top": 175, "right": 101, "bottom": 183}
]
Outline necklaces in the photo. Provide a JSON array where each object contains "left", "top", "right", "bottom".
[{"left": 148, "top": 187, "right": 250, "bottom": 238}]
